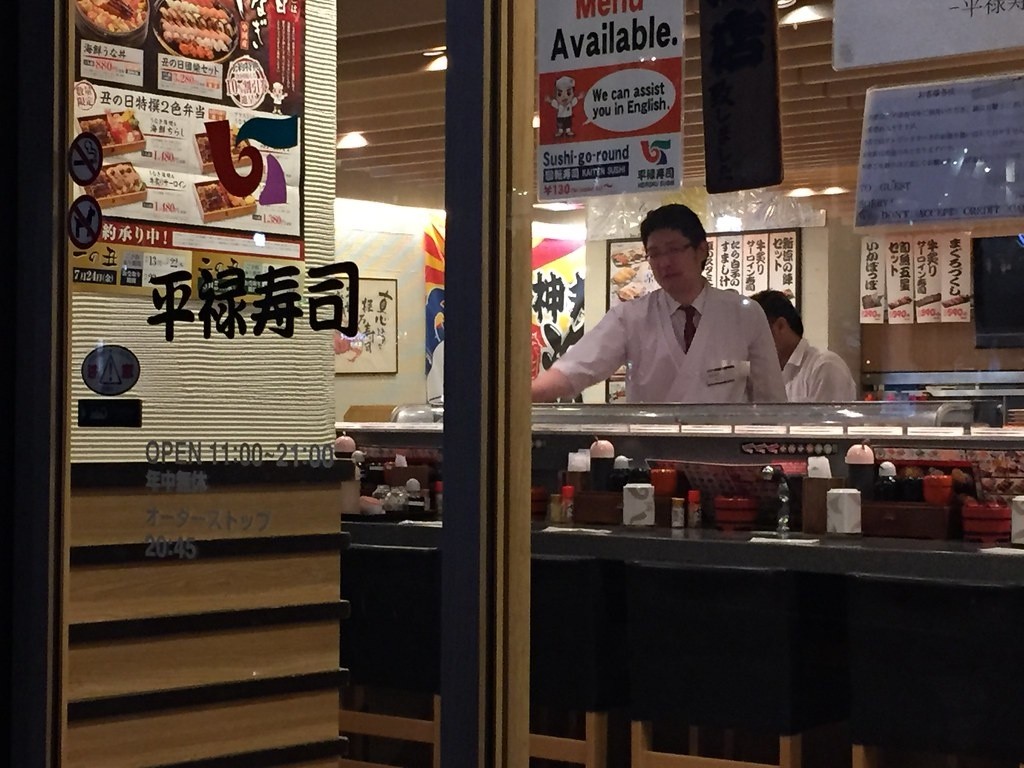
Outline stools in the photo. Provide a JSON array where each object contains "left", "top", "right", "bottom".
[
  {"left": 620, "top": 559, "right": 840, "bottom": 768},
  {"left": 337, "top": 543, "right": 443, "bottom": 768},
  {"left": 528, "top": 553, "right": 623, "bottom": 768},
  {"left": 843, "top": 575, "right": 1024, "bottom": 768}
]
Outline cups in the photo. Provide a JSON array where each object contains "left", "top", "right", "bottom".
[
  {"left": 827, "top": 488, "right": 861, "bottom": 534},
  {"left": 1011, "top": 495, "right": 1024, "bottom": 545},
  {"left": 923, "top": 475, "right": 953, "bottom": 507},
  {"left": 622, "top": 483, "right": 655, "bottom": 525}
]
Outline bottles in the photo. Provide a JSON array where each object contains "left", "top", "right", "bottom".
[
  {"left": 405, "top": 478, "right": 421, "bottom": 502},
  {"left": 562, "top": 486, "right": 574, "bottom": 523},
  {"left": 551, "top": 494, "right": 561, "bottom": 524},
  {"left": 433, "top": 481, "right": 443, "bottom": 521},
  {"left": 878, "top": 461, "right": 897, "bottom": 501},
  {"left": 613, "top": 455, "right": 634, "bottom": 492},
  {"left": 688, "top": 490, "right": 702, "bottom": 528},
  {"left": 671, "top": 497, "right": 685, "bottom": 528},
  {"left": 590, "top": 439, "right": 615, "bottom": 491},
  {"left": 844, "top": 444, "right": 875, "bottom": 493},
  {"left": 334, "top": 431, "right": 356, "bottom": 481}
]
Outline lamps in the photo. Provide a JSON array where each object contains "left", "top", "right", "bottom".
[
  {"left": 336, "top": 131, "right": 369, "bottom": 150},
  {"left": 777, "top": 0, "right": 833, "bottom": 30},
  {"left": 423, "top": 52, "right": 448, "bottom": 72}
]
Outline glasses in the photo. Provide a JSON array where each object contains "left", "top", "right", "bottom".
[{"left": 645, "top": 241, "right": 693, "bottom": 267}]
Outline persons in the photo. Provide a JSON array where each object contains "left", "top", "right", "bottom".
[
  {"left": 751, "top": 289, "right": 858, "bottom": 402},
  {"left": 532, "top": 203, "right": 788, "bottom": 402}
]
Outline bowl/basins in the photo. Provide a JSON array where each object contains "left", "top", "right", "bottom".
[
  {"left": 149, "top": 0, "right": 240, "bottom": 63},
  {"left": 74, "top": 0, "right": 150, "bottom": 48}
]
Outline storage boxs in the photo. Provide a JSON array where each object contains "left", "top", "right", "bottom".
[
  {"left": 801, "top": 476, "right": 1024, "bottom": 547},
  {"left": 572, "top": 457, "right": 678, "bottom": 528}
]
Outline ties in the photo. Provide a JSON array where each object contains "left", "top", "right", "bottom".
[{"left": 676, "top": 305, "right": 696, "bottom": 355}]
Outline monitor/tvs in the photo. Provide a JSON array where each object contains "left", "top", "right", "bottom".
[{"left": 973, "top": 234, "right": 1024, "bottom": 349}]
